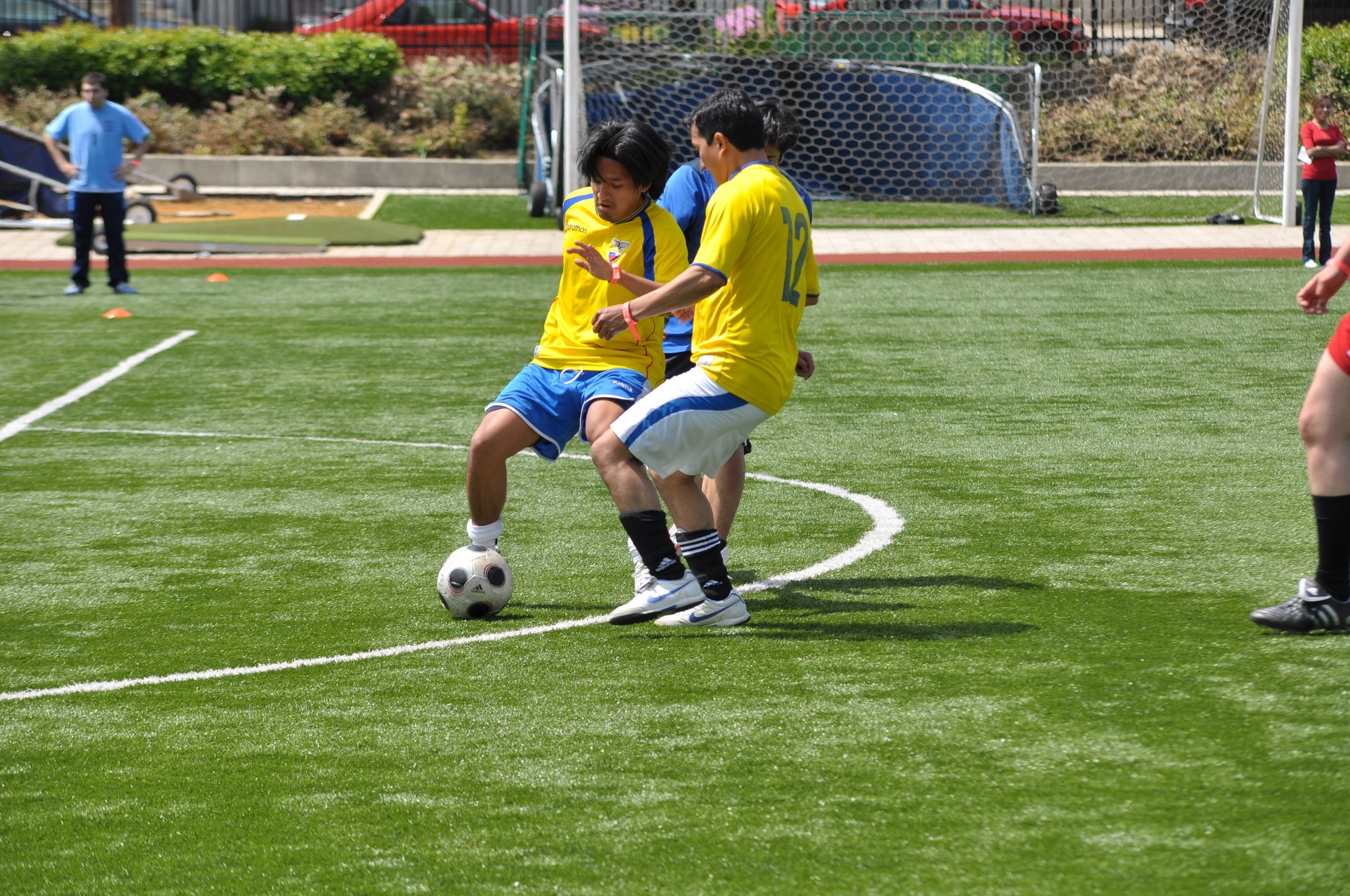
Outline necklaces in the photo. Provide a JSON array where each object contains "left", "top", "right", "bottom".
[{"left": 1319, "top": 128, "right": 1328, "bottom": 138}]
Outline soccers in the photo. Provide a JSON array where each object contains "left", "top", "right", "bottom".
[{"left": 437, "top": 544, "right": 515, "bottom": 621}]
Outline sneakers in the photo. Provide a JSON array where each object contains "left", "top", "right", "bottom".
[
  {"left": 610, "top": 569, "right": 707, "bottom": 625},
  {"left": 1250, "top": 578, "right": 1350, "bottom": 634},
  {"left": 654, "top": 589, "right": 751, "bottom": 626},
  {"left": 668, "top": 522, "right": 684, "bottom": 558},
  {"left": 632, "top": 556, "right": 656, "bottom": 595}
]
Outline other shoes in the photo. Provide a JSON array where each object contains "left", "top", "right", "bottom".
[
  {"left": 1304, "top": 260, "right": 1319, "bottom": 269},
  {"left": 64, "top": 282, "right": 83, "bottom": 295},
  {"left": 115, "top": 283, "right": 137, "bottom": 293}
]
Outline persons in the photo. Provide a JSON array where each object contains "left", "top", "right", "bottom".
[
  {"left": 1301, "top": 95, "right": 1348, "bottom": 267},
  {"left": 42, "top": 72, "right": 153, "bottom": 295},
  {"left": 590, "top": 87, "right": 819, "bottom": 630},
  {"left": 465, "top": 118, "right": 690, "bottom": 595},
  {"left": 1251, "top": 241, "right": 1350, "bottom": 634},
  {"left": 652, "top": 97, "right": 815, "bottom": 565}
]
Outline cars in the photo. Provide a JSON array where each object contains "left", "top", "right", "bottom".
[
  {"left": 289, "top": 0, "right": 612, "bottom": 69},
  {"left": 793, "top": 0, "right": 1093, "bottom": 73},
  {"left": 0, "top": 0, "right": 185, "bottom": 37},
  {"left": 1158, "top": 0, "right": 1348, "bottom": 54}
]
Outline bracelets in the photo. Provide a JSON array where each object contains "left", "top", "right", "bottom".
[
  {"left": 1325, "top": 258, "right": 1350, "bottom": 276},
  {"left": 129, "top": 160, "right": 142, "bottom": 166},
  {"left": 623, "top": 300, "right": 640, "bottom": 341},
  {"left": 608, "top": 262, "right": 620, "bottom": 284}
]
